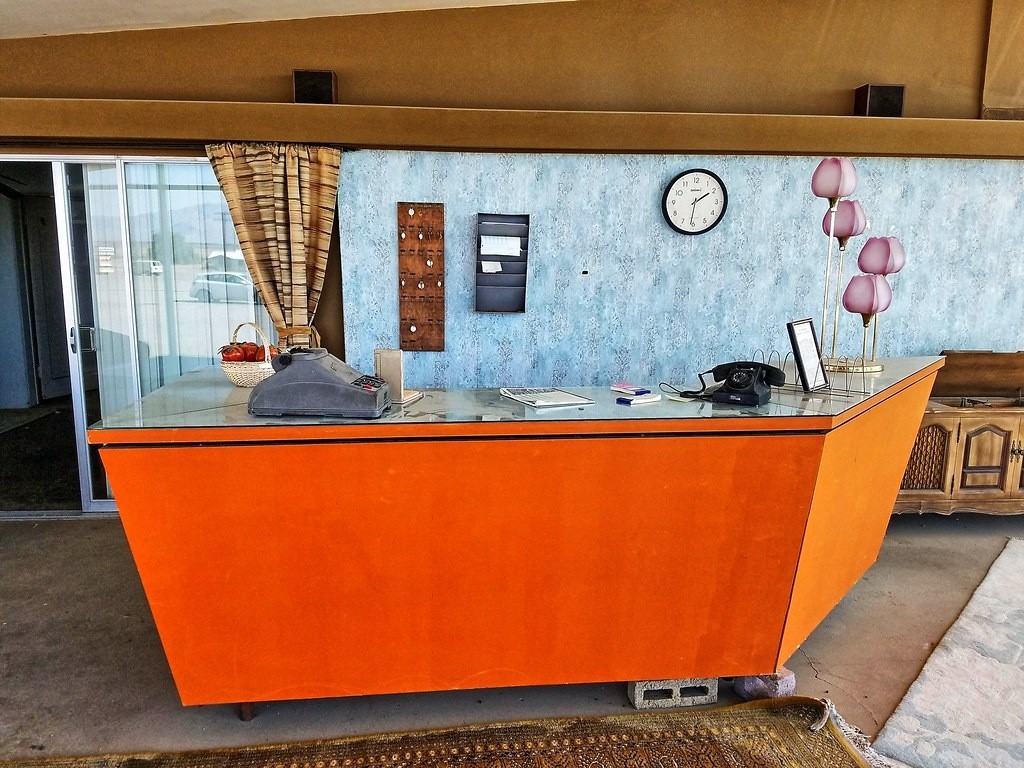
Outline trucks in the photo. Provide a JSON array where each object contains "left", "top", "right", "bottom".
[{"left": 94, "top": 247, "right": 115, "bottom": 275}]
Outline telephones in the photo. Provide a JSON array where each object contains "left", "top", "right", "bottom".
[{"left": 712, "top": 361, "right": 786, "bottom": 406}]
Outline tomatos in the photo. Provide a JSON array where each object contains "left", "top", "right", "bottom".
[{"left": 222, "top": 342, "right": 278, "bottom": 362}]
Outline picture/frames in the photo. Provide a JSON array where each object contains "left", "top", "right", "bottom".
[{"left": 786, "top": 318, "right": 830, "bottom": 394}]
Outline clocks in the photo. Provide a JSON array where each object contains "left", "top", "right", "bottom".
[{"left": 661, "top": 168, "right": 728, "bottom": 235}]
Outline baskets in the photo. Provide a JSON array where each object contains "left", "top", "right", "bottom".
[{"left": 220, "top": 322, "right": 276, "bottom": 388}]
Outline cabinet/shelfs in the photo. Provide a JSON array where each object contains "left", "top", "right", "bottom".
[
  {"left": 891, "top": 416, "right": 959, "bottom": 518},
  {"left": 952, "top": 417, "right": 1023, "bottom": 516}
]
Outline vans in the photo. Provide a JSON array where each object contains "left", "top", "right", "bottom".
[
  {"left": 189, "top": 272, "right": 264, "bottom": 305},
  {"left": 132, "top": 260, "right": 164, "bottom": 276}
]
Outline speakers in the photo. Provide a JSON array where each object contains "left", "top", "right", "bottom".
[
  {"left": 853, "top": 83, "right": 905, "bottom": 118},
  {"left": 291, "top": 70, "right": 337, "bottom": 105}
]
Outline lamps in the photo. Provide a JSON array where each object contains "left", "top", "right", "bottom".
[{"left": 809, "top": 156, "right": 906, "bottom": 374}]
